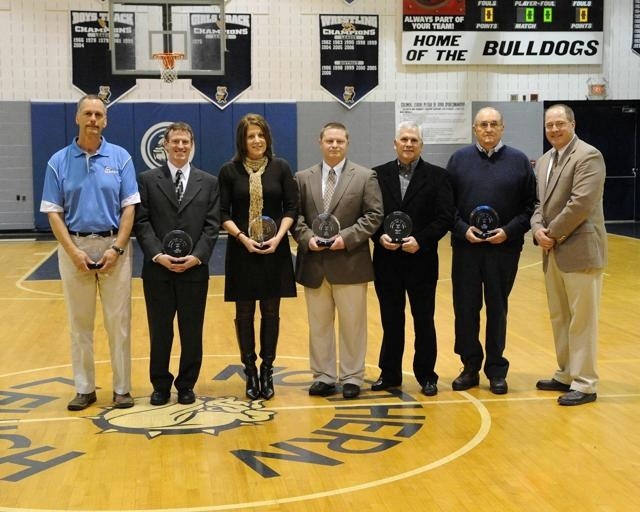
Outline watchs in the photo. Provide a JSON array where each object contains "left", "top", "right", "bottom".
[{"left": 112, "top": 245, "right": 126, "bottom": 256}]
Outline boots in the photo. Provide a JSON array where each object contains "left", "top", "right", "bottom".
[
  {"left": 234, "top": 319, "right": 260, "bottom": 400},
  {"left": 260, "top": 318, "right": 279, "bottom": 400}
]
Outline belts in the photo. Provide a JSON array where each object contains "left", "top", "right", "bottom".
[{"left": 69, "top": 228, "right": 118, "bottom": 237}]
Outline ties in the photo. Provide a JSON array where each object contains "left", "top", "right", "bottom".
[
  {"left": 324, "top": 169, "right": 337, "bottom": 212},
  {"left": 174, "top": 170, "right": 183, "bottom": 205},
  {"left": 548, "top": 150, "right": 558, "bottom": 184}
]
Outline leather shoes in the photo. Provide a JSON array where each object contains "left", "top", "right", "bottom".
[
  {"left": 490, "top": 377, "right": 508, "bottom": 394},
  {"left": 343, "top": 383, "right": 360, "bottom": 398},
  {"left": 372, "top": 377, "right": 401, "bottom": 390},
  {"left": 309, "top": 381, "right": 335, "bottom": 396},
  {"left": 536, "top": 378, "right": 570, "bottom": 391},
  {"left": 423, "top": 380, "right": 437, "bottom": 396},
  {"left": 557, "top": 390, "right": 597, "bottom": 406},
  {"left": 178, "top": 389, "right": 195, "bottom": 404},
  {"left": 452, "top": 372, "right": 479, "bottom": 390},
  {"left": 150, "top": 390, "right": 170, "bottom": 405}
]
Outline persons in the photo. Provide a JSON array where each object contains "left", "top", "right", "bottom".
[
  {"left": 133, "top": 122, "right": 221, "bottom": 405},
  {"left": 530, "top": 104, "right": 609, "bottom": 407},
  {"left": 218, "top": 114, "right": 299, "bottom": 401},
  {"left": 38, "top": 94, "right": 143, "bottom": 410},
  {"left": 290, "top": 121, "right": 384, "bottom": 400},
  {"left": 446, "top": 105, "right": 537, "bottom": 394},
  {"left": 369, "top": 120, "right": 454, "bottom": 396}
]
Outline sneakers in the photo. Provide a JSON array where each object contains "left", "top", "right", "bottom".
[
  {"left": 113, "top": 391, "right": 134, "bottom": 408},
  {"left": 68, "top": 390, "right": 96, "bottom": 410}
]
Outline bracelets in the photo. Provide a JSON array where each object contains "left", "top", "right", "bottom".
[{"left": 235, "top": 231, "right": 244, "bottom": 239}]
